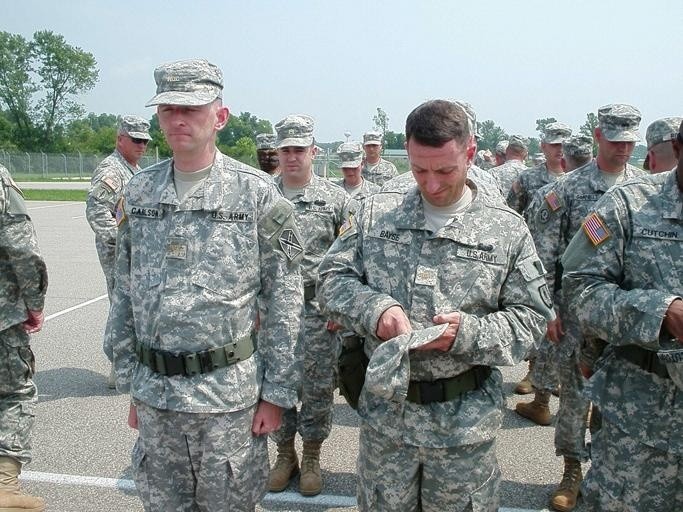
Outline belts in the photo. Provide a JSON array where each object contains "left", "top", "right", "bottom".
[
  {"left": 134, "top": 333, "right": 257, "bottom": 382},
  {"left": 303, "top": 282, "right": 315, "bottom": 304},
  {"left": 365, "top": 357, "right": 492, "bottom": 406},
  {"left": 617, "top": 341, "right": 670, "bottom": 379}
]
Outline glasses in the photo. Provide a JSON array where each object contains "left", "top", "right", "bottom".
[{"left": 121, "top": 134, "right": 149, "bottom": 145}]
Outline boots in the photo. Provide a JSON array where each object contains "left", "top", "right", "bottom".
[
  {"left": 268, "top": 437, "right": 300, "bottom": 492},
  {"left": 298, "top": 442, "right": 325, "bottom": 497},
  {"left": 551, "top": 457, "right": 585, "bottom": 511},
  {"left": 0, "top": 455, "right": 45, "bottom": 511},
  {"left": 513, "top": 358, "right": 592, "bottom": 429}
]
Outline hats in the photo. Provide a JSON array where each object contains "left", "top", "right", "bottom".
[
  {"left": 117, "top": 113, "right": 154, "bottom": 140},
  {"left": 144, "top": 59, "right": 224, "bottom": 109},
  {"left": 356, "top": 320, "right": 453, "bottom": 411},
  {"left": 643, "top": 117, "right": 682, "bottom": 171},
  {"left": 255, "top": 133, "right": 276, "bottom": 150},
  {"left": 449, "top": 99, "right": 595, "bottom": 156},
  {"left": 598, "top": 102, "right": 643, "bottom": 144},
  {"left": 274, "top": 113, "right": 315, "bottom": 149},
  {"left": 336, "top": 141, "right": 364, "bottom": 169},
  {"left": 362, "top": 131, "right": 382, "bottom": 147}
]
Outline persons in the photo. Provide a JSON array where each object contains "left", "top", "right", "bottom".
[{"left": 2, "top": 165, "right": 48, "bottom": 510}]
